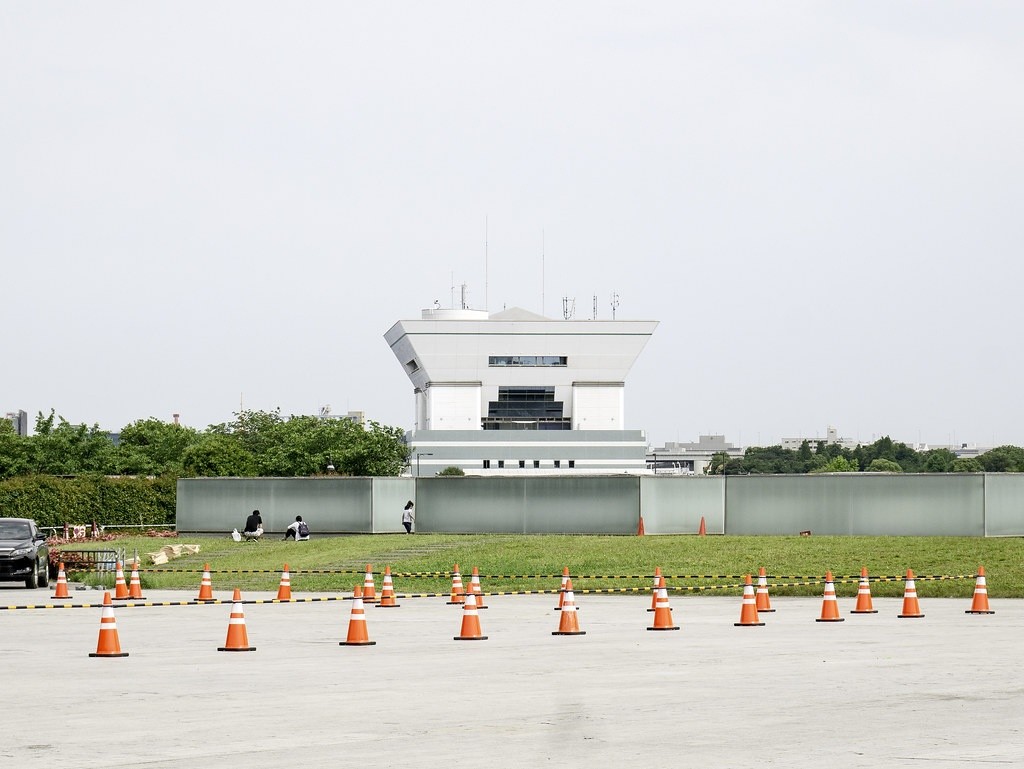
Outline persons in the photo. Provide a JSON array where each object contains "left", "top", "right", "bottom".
[
  {"left": 402, "top": 501, "right": 415, "bottom": 534},
  {"left": 244, "top": 510, "right": 263, "bottom": 542},
  {"left": 282, "top": 516, "right": 309, "bottom": 541}
]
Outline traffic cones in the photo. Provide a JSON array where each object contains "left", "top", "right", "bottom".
[
  {"left": 111, "top": 560, "right": 135, "bottom": 601},
  {"left": 363, "top": 563, "right": 381, "bottom": 603},
  {"left": 551, "top": 579, "right": 586, "bottom": 635},
  {"left": 756, "top": 567, "right": 776, "bottom": 612},
  {"left": 698, "top": 516, "right": 706, "bottom": 536},
  {"left": 339, "top": 584, "right": 376, "bottom": 645},
  {"left": 446, "top": 563, "right": 466, "bottom": 604},
  {"left": 733, "top": 574, "right": 765, "bottom": 626},
  {"left": 271, "top": 563, "right": 291, "bottom": 601},
  {"left": 217, "top": 589, "right": 257, "bottom": 652},
  {"left": 965, "top": 566, "right": 995, "bottom": 614},
  {"left": 453, "top": 581, "right": 489, "bottom": 641},
  {"left": 553, "top": 567, "right": 580, "bottom": 610},
  {"left": 850, "top": 566, "right": 879, "bottom": 614},
  {"left": 646, "top": 576, "right": 680, "bottom": 631},
  {"left": 375, "top": 566, "right": 401, "bottom": 608},
  {"left": 898, "top": 569, "right": 925, "bottom": 618},
  {"left": 636, "top": 516, "right": 645, "bottom": 536},
  {"left": 193, "top": 562, "right": 217, "bottom": 602},
  {"left": 129, "top": 562, "right": 148, "bottom": 600},
  {"left": 462, "top": 567, "right": 488, "bottom": 610},
  {"left": 814, "top": 569, "right": 845, "bottom": 622},
  {"left": 647, "top": 567, "right": 672, "bottom": 612},
  {"left": 88, "top": 591, "right": 129, "bottom": 657},
  {"left": 50, "top": 561, "right": 73, "bottom": 599}
]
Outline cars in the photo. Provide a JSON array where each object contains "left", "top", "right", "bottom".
[{"left": 0, "top": 517, "right": 51, "bottom": 589}]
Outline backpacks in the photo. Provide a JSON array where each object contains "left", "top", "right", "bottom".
[{"left": 297, "top": 522, "right": 309, "bottom": 536}]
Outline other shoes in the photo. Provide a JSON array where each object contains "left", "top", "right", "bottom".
[
  {"left": 253, "top": 538, "right": 258, "bottom": 542},
  {"left": 281, "top": 538, "right": 287, "bottom": 541}
]
